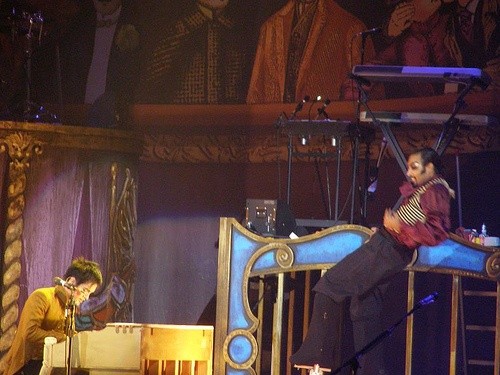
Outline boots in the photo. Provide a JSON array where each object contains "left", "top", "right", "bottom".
[
  {"left": 289, "top": 291, "right": 340, "bottom": 369},
  {"left": 352, "top": 317, "right": 384, "bottom": 375}
]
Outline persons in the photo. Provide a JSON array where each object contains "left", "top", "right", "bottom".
[
  {"left": 289, "top": 146, "right": 456, "bottom": 375},
  {"left": 4, "top": 257, "right": 103, "bottom": 374}
]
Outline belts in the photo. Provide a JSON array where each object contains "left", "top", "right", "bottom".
[{"left": 381, "top": 225, "right": 415, "bottom": 253}]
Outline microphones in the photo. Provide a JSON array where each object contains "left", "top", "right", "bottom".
[
  {"left": 291, "top": 96, "right": 310, "bottom": 120},
  {"left": 416, "top": 292, "right": 439, "bottom": 309},
  {"left": 315, "top": 99, "right": 329, "bottom": 120},
  {"left": 53, "top": 276, "right": 78, "bottom": 291},
  {"left": 356, "top": 27, "right": 385, "bottom": 36}
]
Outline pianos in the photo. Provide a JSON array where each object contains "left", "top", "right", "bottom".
[
  {"left": 352, "top": 63, "right": 497, "bottom": 211},
  {"left": 280, "top": 118, "right": 376, "bottom": 221},
  {"left": 38, "top": 323, "right": 214, "bottom": 375}
]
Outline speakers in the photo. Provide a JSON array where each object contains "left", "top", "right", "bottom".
[{"left": 246, "top": 198, "right": 296, "bottom": 237}]
